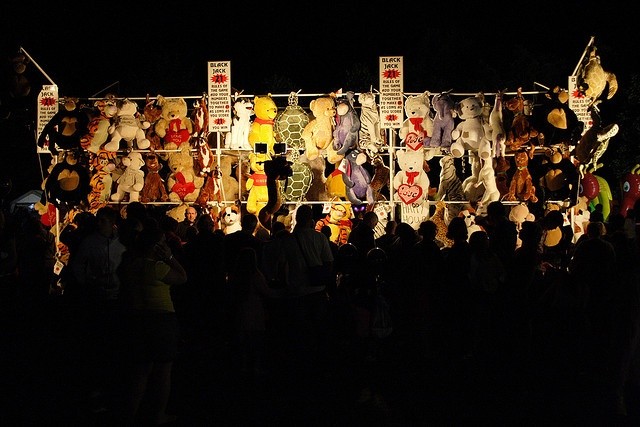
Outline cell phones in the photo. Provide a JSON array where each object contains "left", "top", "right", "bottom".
[{"left": 152, "top": 244, "right": 162, "bottom": 252}]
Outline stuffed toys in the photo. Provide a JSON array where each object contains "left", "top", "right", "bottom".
[
  {"left": 2, "top": 51, "right": 37, "bottom": 126},
  {"left": 41, "top": 42, "right": 621, "bottom": 262},
  {"left": 578, "top": 161, "right": 640, "bottom": 219},
  {"left": 41, "top": 41, "right": 620, "bottom": 264}
]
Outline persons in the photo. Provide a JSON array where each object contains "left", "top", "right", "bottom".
[{"left": 1, "top": 198, "right": 640, "bottom": 426}]
